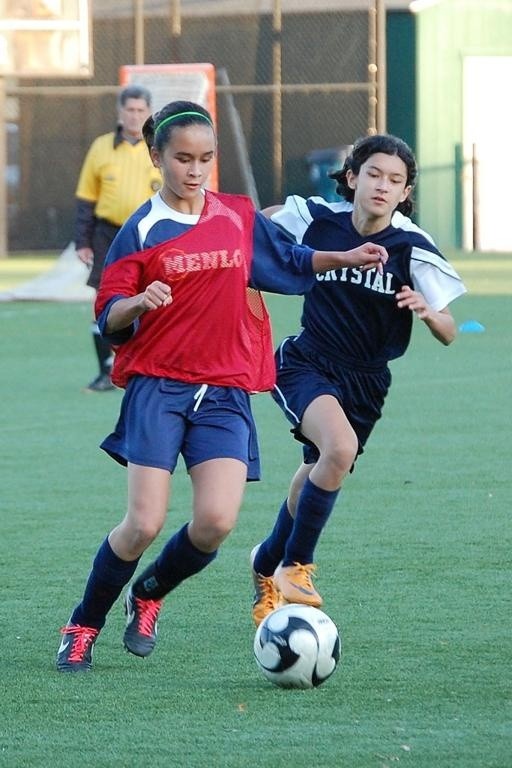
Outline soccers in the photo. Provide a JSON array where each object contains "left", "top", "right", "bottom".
[{"left": 253, "top": 604, "right": 341, "bottom": 689}]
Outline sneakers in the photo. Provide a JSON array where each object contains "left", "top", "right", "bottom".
[
  {"left": 89, "top": 372, "right": 116, "bottom": 389},
  {"left": 56, "top": 543, "right": 323, "bottom": 672}
]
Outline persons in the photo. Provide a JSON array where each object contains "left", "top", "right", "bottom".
[
  {"left": 243, "top": 127, "right": 469, "bottom": 625},
  {"left": 49, "top": 99, "right": 391, "bottom": 678},
  {"left": 70, "top": 86, "right": 162, "bottom": 391}
]
